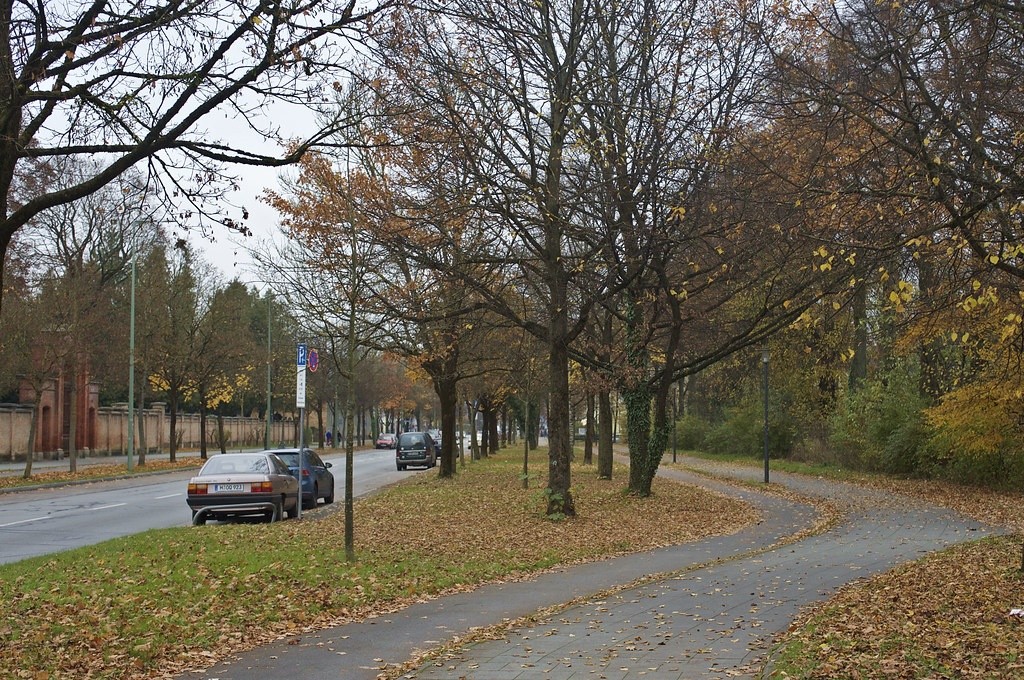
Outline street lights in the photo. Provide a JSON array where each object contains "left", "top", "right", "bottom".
[
  {"left": 760, "top": 346, "right": 773, "bottom": 485},
  {"left": 669, "top": 381, "right": 677, "bottom": 464}
]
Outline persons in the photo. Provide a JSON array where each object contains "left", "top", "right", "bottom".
[
  {"left": 337, "top": 431, "right": 342, "bottom": 444},
  {"left": 325, "top": 430, "right": 331, "bottom": 446}
]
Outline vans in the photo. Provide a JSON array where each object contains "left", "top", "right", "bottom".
[{"left": 396, "top": 431, "right": 438, "bottom": 471}]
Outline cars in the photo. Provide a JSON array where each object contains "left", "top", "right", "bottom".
[
  {"left": 187, "top": 451, "right": 299, "bottom": 525},
  {"left": 574, "top": 427, "right": 586, "bottom": 441},
  {"left": 375, "top": 433, "right": 399, "bottom": 450},
  {"left": 429, "top": 421, "right": 520, "bottom": 459},
  {"left": 259, "top": 447, "right": 334, "bottom": 510}
]
